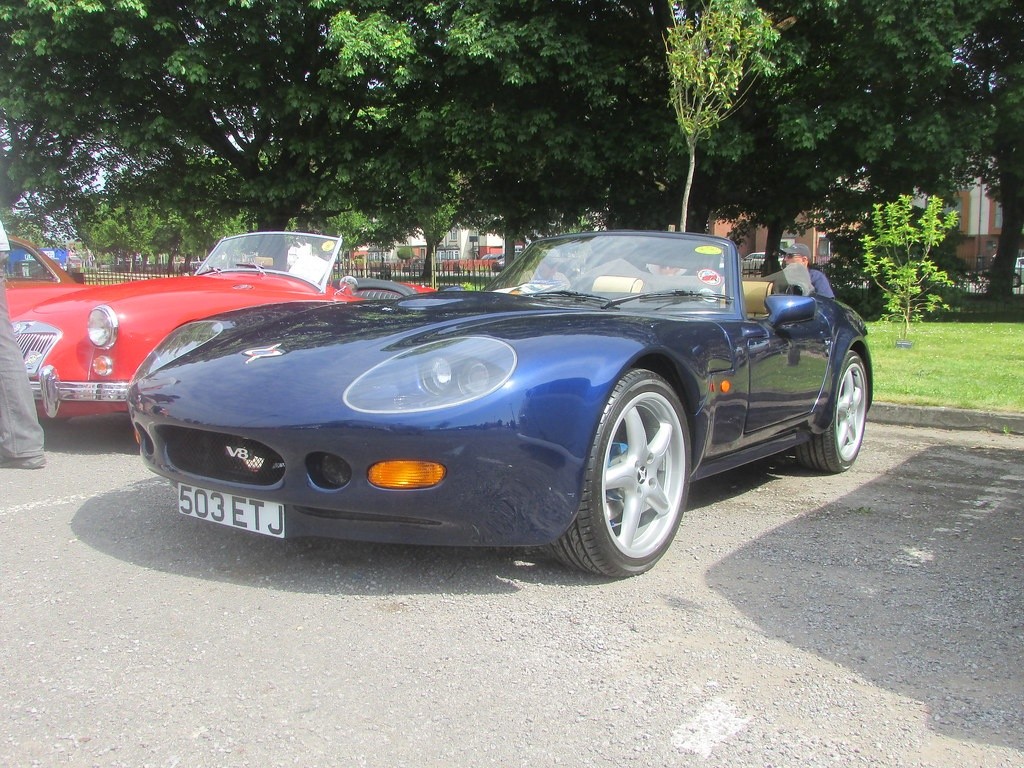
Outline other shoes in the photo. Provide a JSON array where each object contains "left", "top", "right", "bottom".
[{"left": 0, "top": 452, "right": 47, "bottom": 469}]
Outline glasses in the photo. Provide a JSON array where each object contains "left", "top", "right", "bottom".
[{"left": 780, "top": 253, "right": 803, "bottom": 260}]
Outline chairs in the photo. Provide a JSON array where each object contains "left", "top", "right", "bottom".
[
  {"left": 591, "top": 275, "right": 644, "bottom": 294},
  {"left": 720, "top": 280, "right": 773, "bottom": 319}
]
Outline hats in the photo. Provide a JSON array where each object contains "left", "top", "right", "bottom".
[{"left": 779, "top": 244, "right": 811, "bottom": 260}]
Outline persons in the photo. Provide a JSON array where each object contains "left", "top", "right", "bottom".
[
  {"left": 530, "top": 250, "right": 571, "bottom": 291},
  {"left": 779, "top": 244, "right": 835, "bottom": 300},
  {"left": 0, "top": 220, "right": 46, "bottom": 470}
]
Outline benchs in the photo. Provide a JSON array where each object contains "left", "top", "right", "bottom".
[{"left": 631, "top": 274, "right": 727, "bottom": 296}]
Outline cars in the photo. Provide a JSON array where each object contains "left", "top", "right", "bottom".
[
  {"left": 493, "top": 251, "right": 523, "bottom": 271},
  {"left": 1, "top": 235, "right": 77, "bottom": 283},
  {"left": 741, "top": 252, "right": 783, "bottom": 270}
]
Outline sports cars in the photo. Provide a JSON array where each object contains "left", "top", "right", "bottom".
[
  {"left": 127, "top": 230, "right": 875, "bottom": 576},
  {"left": 10, "top": 232, "right": 436, "bottom": 426}
]
